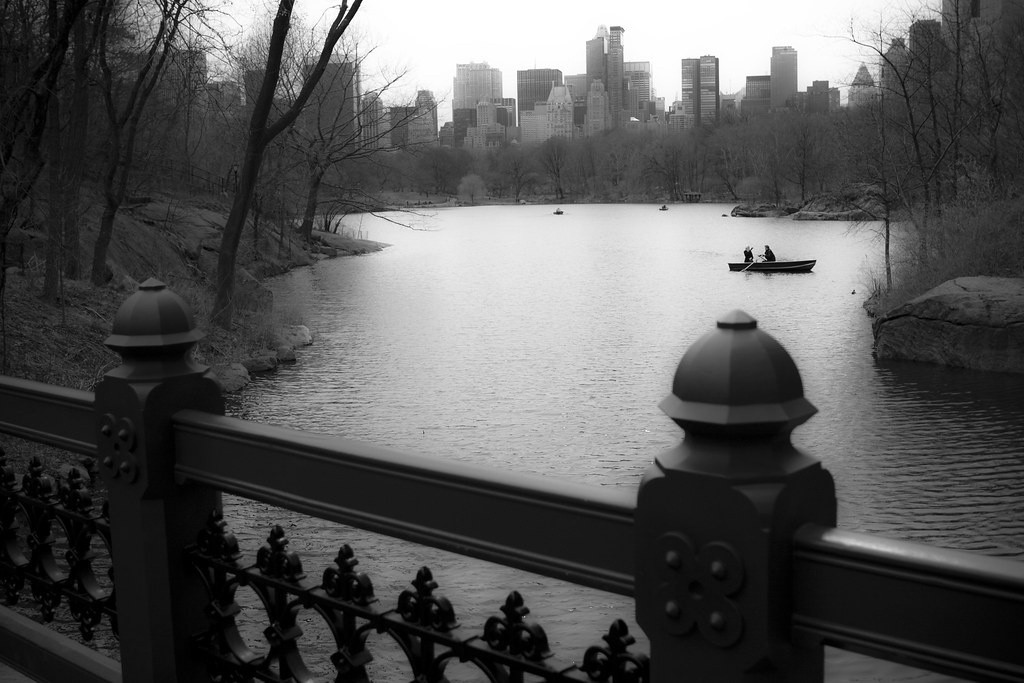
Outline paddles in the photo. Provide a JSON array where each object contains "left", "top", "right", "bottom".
[{"left": 741, "top": 254, "right": 766, "bottom": 272}]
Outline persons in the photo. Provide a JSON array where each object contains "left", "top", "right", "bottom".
[
  {"left": 516, "top": 198, "right": 519, "bottom": 203},
  {"left": 662, "top": 205, "right": 666, "bottom": 208},
  {"left": 744, "top": 247, "right": 754, "bottom": 262},
  {"left": 759, "top": 245, "right": 776, "bottom": 262}
]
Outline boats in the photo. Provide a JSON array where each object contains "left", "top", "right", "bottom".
[
  {"left": 553, "top": 211, "right": 564, "bottom": 215},
  {"left": 659, "top": 208, "right": 668, "bottom": 210},
  {"left": 728, "top": 260, "right": 817, "bottom": 273}
]
